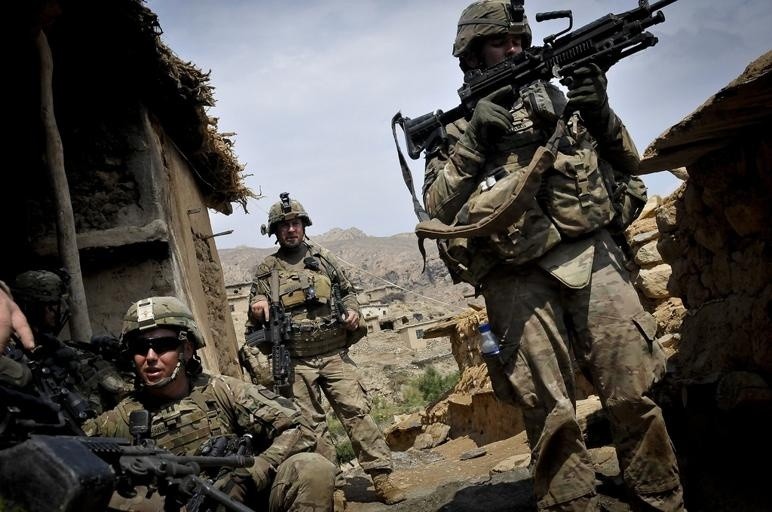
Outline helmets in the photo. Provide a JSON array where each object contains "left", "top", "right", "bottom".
[
  {"left": 260, "top": 190, "right": 313, "bottom": 238},
  {"left": 10, "top": 270, "right": 72, "bottom": 306},
  {"left": 116, "top": 295, "right": 210, "bottom": 352},
  {"left": 453, "top": 1, "right": 536, "bottom": 61}
]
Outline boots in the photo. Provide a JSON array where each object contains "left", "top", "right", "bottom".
[
  {"left": 333, "top": 487, "right": 350, "bottom": 510},
  {"left": 369, "top": 469, "right": 406, "bottom": 505}
]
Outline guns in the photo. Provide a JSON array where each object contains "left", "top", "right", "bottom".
[
  {"left": 402, "top": 1, "right": 677, "bottom": 160},
  {"left": 1, "top": 433, "right": 257, "bottom": 511},
  {"left": 246, "top": 301, "right": 293, "bottom": 400},
  {"left": 0, "top": 340, "right": 133, "bottom": 433}
]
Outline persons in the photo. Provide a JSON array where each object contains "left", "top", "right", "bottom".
[
  {"left": 246, "top": 199, "right": 409, "bottom": 511},
  {"left": 82, "top": 296, "right": 336, "bottom": 511},
  {"left": 423, "top": 0, "right": 687, "bottom": 511},
  {"left": 8, "top": 269, "right": 133, "bottom": 414},
  {"left": 1, "top": 282, "right": 96, "bottom": 440}
]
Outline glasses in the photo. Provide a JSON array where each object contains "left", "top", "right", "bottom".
[{"left": 129, "top": 335, "right": 186, "bottom": 357}]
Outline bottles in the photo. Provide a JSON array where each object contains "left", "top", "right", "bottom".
[{"left": 476, "top": 322, "right": 502, "bottom": 358}]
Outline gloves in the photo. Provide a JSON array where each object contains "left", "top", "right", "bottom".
[
  {"left": 465, "top": 83, "right": 517, "bottom": 144},
  {"left": 563, "top": 65, "right": 608, "bottom": 113}
]
